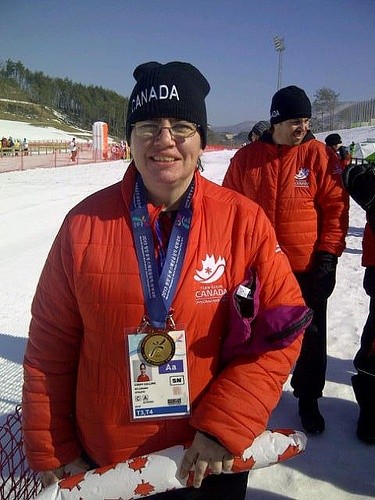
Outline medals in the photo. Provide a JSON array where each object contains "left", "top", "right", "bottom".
[{"left": 141, "top": 331, "right": 175, "bottom": 367}]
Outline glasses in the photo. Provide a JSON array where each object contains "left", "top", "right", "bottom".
[{"left": 130, "top": 120, "right": 200, "bottom": 138}]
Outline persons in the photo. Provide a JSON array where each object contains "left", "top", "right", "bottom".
[
  {"left": 221, "top": 85, "right": 350, "bottom": 435},
  {"left": 342, "top": 163, "right": 375, "bottom": 443},
  {"left": 21, "top": 61, "right": 314, "bottom": 500},
  {"left": 350, "top": 141, "right": 356, "bottom": 160},
  {"left": 0, "top": 136, "right": 28, "bottom": 157},
  {"left": 325, "top": 133, "right": 352, "bottom": 173},
  {"left": 70, "top": 138, "right": 78, "bottom": 162},
  {"left": 247, "top": 120, "right": 271, "bottom": 142},
  {"left": 137, "top": 363, "right": 151, "bottom": 382}
]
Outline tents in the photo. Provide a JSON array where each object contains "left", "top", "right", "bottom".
[{"left": 352, "top": 143, "right": 375, "bottom": 162}]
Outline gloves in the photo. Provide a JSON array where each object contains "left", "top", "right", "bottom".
[
  {"left": 223, "top": 267, "right": 259, "bottom": 350},
  {"left": 310, "top": 252, "right": 337, "bottom": 306},
  {"left": 342, "top": 164, "right": 375, "bottom": 216},
  {"left": 340, "top": 146, "right": 348, "bottom": 158},
  {"left": 222, "top": 306, "right": 313, "bottom": 361}
]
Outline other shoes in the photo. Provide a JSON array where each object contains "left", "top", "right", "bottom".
[
  {"left": 357, "top": 414, "right": 375, "bottom": 444},
  {"left": 299, "top": 400, "right": 325, "bottom": 433}
]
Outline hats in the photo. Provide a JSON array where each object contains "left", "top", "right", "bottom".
[
  {"left": 325, "top": 134, "right": 342, "bottom": 144},
  {"left": 270, "top": 86, "right": 311, "bottom": 124},
  {"left": 248, "top": 121, "right": 271, "bottom": 141},
  {"left": 126, "top": 61, "right": 211, "bottom": 150}
]
